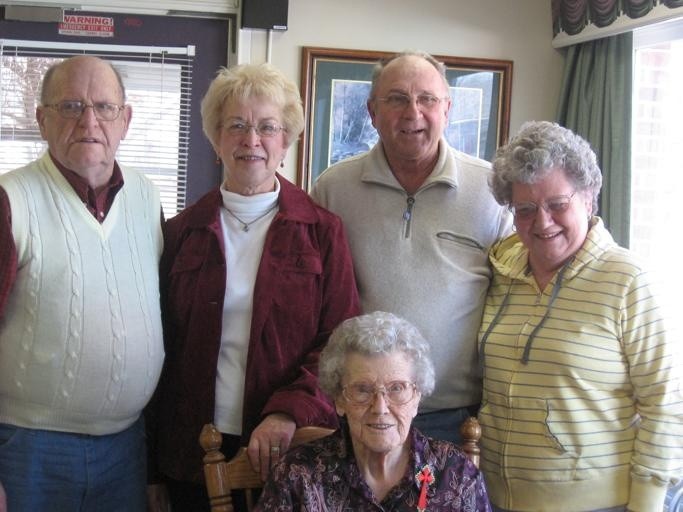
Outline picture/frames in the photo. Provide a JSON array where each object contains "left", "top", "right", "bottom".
[{"left": 291, "top": 43, "right": 513, "bottom": 199}]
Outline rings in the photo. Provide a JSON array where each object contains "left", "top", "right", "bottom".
[{"left": 270, "top": 446, "right": 280, "bottom": 452}]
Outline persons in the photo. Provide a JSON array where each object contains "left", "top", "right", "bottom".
[
  {"left": 142, "top": 63, "right": 362, "bottom": 511},
  {"left": 303, "top": 49, "right": 516, "bottom": 453},
  {"left": 1, "top": 56, "right": 167, "bottom": 512},
  {"left": 255, "top": 305, "right": 492, "bottom": 512},
  {"left": 474, "top": 121, "right": 681, "bottom": 511}
]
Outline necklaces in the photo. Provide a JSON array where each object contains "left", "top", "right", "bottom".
[{"left": 220, "top": 204, "right": 280, "bottom": 234}]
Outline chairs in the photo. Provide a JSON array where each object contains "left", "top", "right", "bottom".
[{"left": 196, "top": 409, "right": 481, "bottom": 512}]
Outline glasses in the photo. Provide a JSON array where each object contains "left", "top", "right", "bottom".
[
  {"left": 507, "top": 188, "right": 579, "bottom": 218},
  {"left": 41, "top": 100, "right": 125, "bottom": 121},
  {"left": 215, "top": 118, "right": 286, "bottom": 137},
  {"left": 372, "top": 92, "right": 451, "bottom": 113},
  {"left": 338, "top": 379, "right": 418, "bottom": 406}
]
